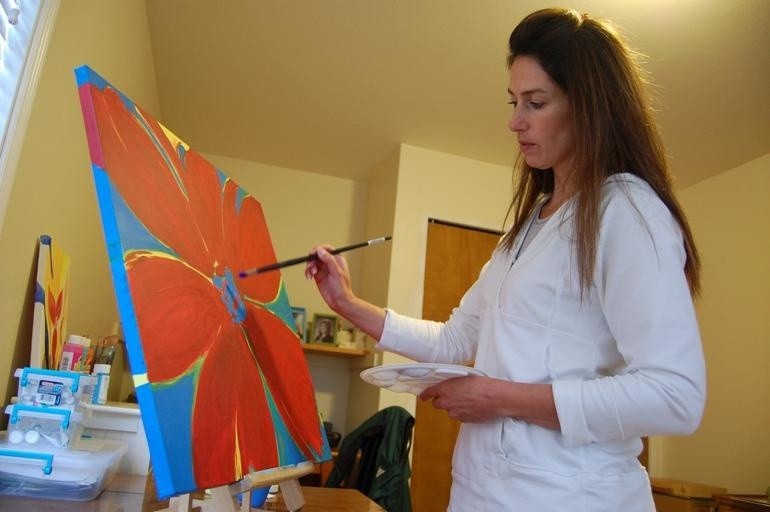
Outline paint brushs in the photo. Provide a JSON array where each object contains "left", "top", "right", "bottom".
[{"left": 238, "top": 236, "right": 394, "bottom": 278}]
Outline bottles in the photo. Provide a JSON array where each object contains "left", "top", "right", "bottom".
[
  {"left": 305, "top": 321, "right": 312, "bottom": 344},
  {"left": 67, "top": 394, "right": 90, "bottom": 450},
  {"left": 336, "top": 327, "right": 366, "bottom": 349},
  {"left": 59, "top": 334, "right": 118, "bottom": 404}
]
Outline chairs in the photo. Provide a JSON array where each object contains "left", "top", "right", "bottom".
[{"left": 331, "top": 405, "right": 416, "bottom": 511}]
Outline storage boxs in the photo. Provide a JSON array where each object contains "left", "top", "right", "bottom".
[{"left": 0, "top": 363, "right": 128, "bottom": 501}]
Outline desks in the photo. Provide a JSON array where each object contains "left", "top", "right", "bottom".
[{"left": 2, "top": 397, "right": 156, "bottom": 512}]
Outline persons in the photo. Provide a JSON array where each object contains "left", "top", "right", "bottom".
[
  {"left": 304, "top": 10, "right": 707, "bottom": 512},
  {"left": 315, "top": 320, "right": 335, "bottom": 342}
]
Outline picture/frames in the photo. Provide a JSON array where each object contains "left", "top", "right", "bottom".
[{"left": 292, "top": 305, "right": 340, "bottom": 346}]
